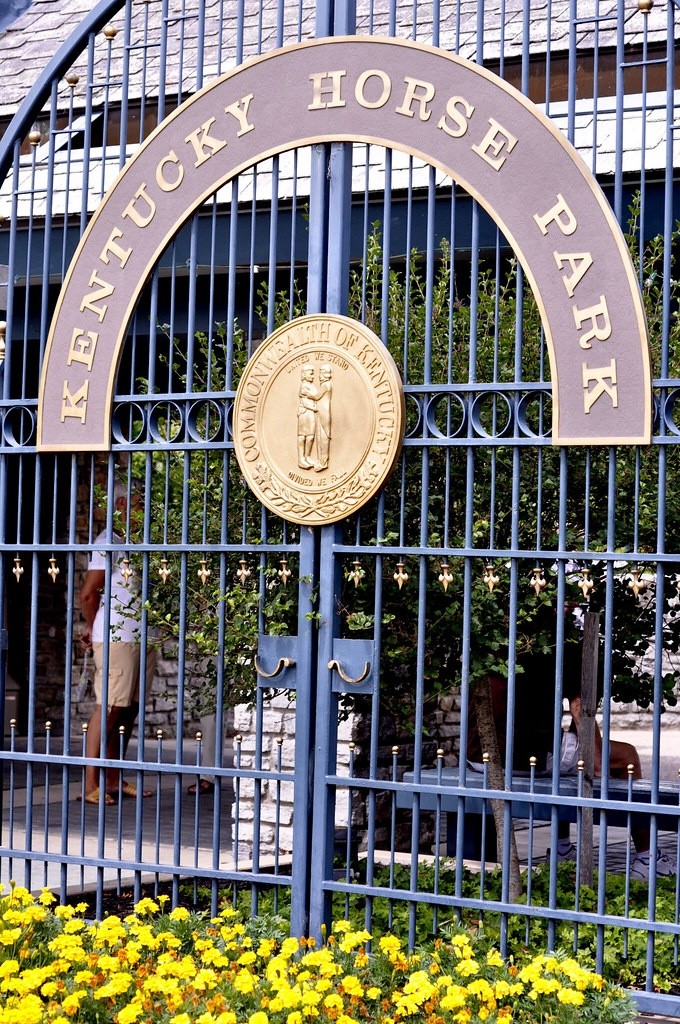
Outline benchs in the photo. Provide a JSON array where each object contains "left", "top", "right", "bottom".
[{"left": 398, "top": 765, "right": 680, "bottom": 860}]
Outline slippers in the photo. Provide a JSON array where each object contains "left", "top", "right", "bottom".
[
  {"left": 76, "top": 787, "right": 116, "bottom": 806},
  {"left": 107, "top": 783, "right": 155, "bottom": 800}
]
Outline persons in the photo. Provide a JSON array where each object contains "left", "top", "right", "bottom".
[
  {"left": 187, "top": 656, "right": 230, "bottom": 795},
  {"left": 467, "top": 582, "right": 677, "bottom": 879},
  {"left": 297, "top": 366, "right": 333, "bottom": 472},
  {"left": 78, "top": 480, "right": 161, "bottom": 805}
]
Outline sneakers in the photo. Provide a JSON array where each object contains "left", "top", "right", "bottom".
[
  {"left": 626, "top": 847, "right": 680, "bottom": 880},
  {"left": 546, "top": 843, "right": 577, "bottom": 867}
]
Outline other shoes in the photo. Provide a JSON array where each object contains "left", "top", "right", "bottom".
[{"left": 187, "top": 779, "right": 215, "bottom": 796}]
